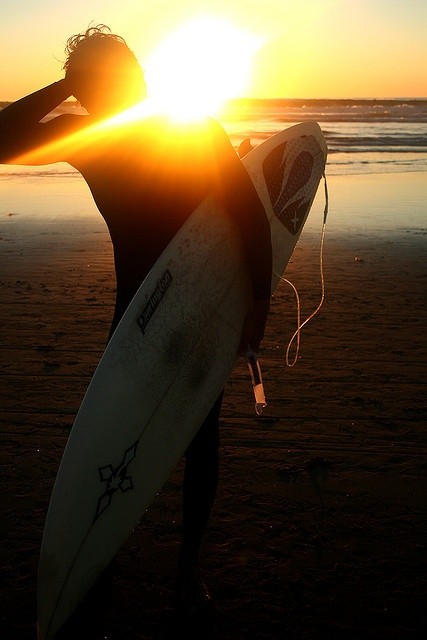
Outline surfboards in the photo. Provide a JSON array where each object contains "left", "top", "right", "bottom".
[{"left": 36, "top": 120, "right": 325, "bottom": 636}]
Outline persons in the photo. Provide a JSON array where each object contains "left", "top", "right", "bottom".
[{"left": 0, "top": 20, "right": 271, "bottom": 605}]
